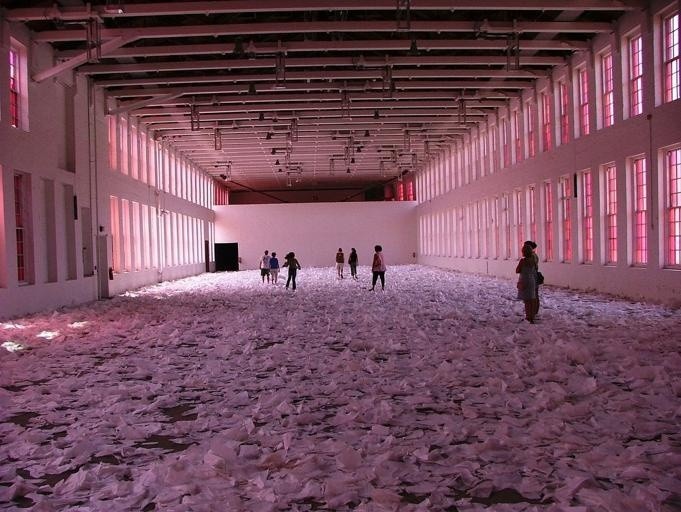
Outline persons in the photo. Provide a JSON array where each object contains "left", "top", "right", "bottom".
[
  {"left": 283, "top": 252, "right": 302, "bottom": 292},
  {"left": 516, "top": 244, "right": 539, "bottom": 324},
  {"left": 348, "top": 246, "right": 359, "bottom": 279},
  {"left": 336, "top": 247, "right": 345, "bottom": 280},
  {"left": 269, "top": 251, "right": 281, "bottom": 284},
  {"left": 370, "top": 243, "right": 388, "bottom": 292},
  {"left": 523, "top": 240, "right": 540, "bottom": 314},
  {"left": 260, "top": 250, "right": 272, "bottom": 284}
]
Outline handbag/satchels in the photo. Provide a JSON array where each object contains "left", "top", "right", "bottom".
[{"left": 535, "top": 272, "right": 544, "bottom": 285}]
